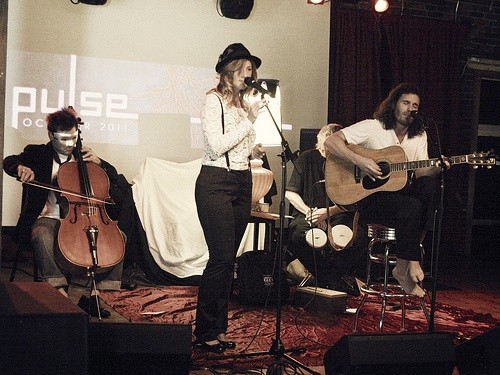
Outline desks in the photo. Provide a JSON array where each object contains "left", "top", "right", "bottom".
[{"left": 248, "top": 212, "right": 280, "bottom": 252}]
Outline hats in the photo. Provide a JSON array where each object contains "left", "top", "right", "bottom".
[{"left": 215, "top": 42, "right": 262, "bottom": 73}]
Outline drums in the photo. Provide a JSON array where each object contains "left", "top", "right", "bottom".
[
  {"left": 331, "top": 224, "right": 354, "bottom": 249},
  {"left": 305, "top": 227, "right": 327, "bottom": 249}
]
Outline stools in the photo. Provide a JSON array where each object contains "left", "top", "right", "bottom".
[{"left": 355, "top": 224, "right": 430, "bottom": 332}]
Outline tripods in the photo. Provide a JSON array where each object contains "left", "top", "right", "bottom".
[{"left": 206, "top": 91, "right": 323, "bottom": 375}]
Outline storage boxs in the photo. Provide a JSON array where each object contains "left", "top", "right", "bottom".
[{"left": 295, "top": 287, "right": 347, "bottom": 314}]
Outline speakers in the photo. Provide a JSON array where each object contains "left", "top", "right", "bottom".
[
  {"left": 454, "top": 326, "right": 500, "bottom": 375},
  {"left": 323, "top": 332, "right": 456, "bottom": 375},
  {"left": 0, "top": 314, "right": 89, "bottom": 375},
  {"left": 86, "top": 322, "right": 193, "bottom": 374}
]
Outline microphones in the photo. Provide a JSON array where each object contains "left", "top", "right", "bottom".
[
  {"left": 411, "top": 110, "right": 431, "bottom": 125},
  {"left": 245, "top": 77, "right": 272, "bottom": 96}
]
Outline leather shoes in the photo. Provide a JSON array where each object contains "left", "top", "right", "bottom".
[
  {"left": 216, "top": 335, "right": 236, "bottom": 349},
  {"left": 195, "top": 337, "right": 225, "bottom": 354}
]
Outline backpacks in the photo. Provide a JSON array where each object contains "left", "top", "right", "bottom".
[{"left": 235, "top": 250, "right": 290, "bottom": 307}]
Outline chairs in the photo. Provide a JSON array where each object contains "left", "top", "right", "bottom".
[{"left": 9, "top": 236, "right": 39, "bottom": 281}]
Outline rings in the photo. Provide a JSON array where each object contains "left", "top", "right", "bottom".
[{"left": 23, "top": 170, "right": 27, "bottom": 172}]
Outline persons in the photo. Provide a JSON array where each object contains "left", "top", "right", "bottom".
[
  {"left": 194, "top": 41, "right": 266, "bottom": 351},
  {"left": 325, "top": 81, "right": 450, "bottom": 299},
  {"left": 285, "top": 122, "right": 359, "bottom": 297},
  {"left": 3, "top": 110, "right": 119, "bottom": 319}
]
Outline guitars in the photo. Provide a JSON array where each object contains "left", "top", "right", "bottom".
[{"left": 325, "top": 143, "right": 500, "bottom": 215}]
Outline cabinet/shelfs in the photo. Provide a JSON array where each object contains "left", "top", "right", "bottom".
[{"left": 459, "top": 58, "right": 500, "bottom": 257}]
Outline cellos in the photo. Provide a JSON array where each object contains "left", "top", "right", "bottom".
[{"left": 50, "top": 102, "right": 127, "bottom": 321}]
